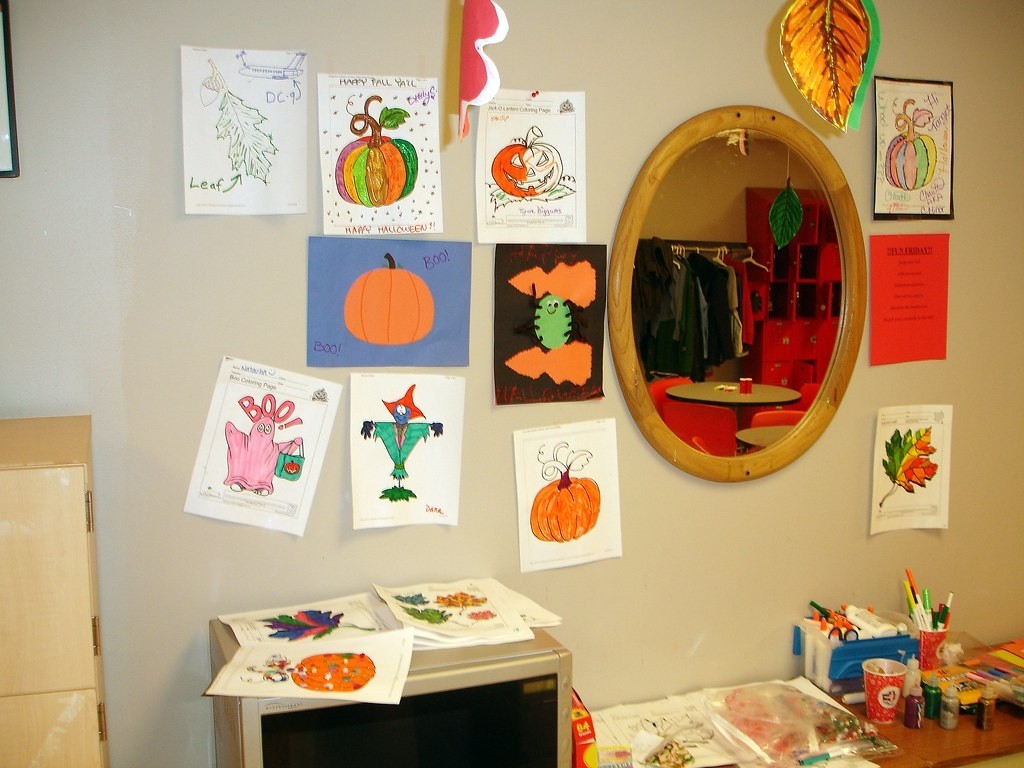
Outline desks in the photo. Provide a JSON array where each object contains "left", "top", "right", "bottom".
[{"left": 666, "top": 382, "right": 801, "bottom": 450}]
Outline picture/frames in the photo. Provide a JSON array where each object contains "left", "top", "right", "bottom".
[{"left": 872, "top": 75, "right": 955, "bottom": 220}]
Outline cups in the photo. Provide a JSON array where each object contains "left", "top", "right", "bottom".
[
  {"left": 862, "top": 658, "right": 907, "bottom": 724},
  {"left": 917, "top": 625, "right": 949, "bottom": 671}
]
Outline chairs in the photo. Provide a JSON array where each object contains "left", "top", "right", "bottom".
[{"left": 650, "top": 378, "right": 821, "bottom": 456}]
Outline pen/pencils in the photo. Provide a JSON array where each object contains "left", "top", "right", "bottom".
[
  {"left": 801, "top": 746, "right": 850, "bottom": 766},
  {"left": 810, "top": 600, "right": 829, "bottom": 618},
  {"left": 903, "top": 567, "right": 954, "bottom": 632},
  {"left": 965, "top": 666, "right": 1024, "bottom": 707}
]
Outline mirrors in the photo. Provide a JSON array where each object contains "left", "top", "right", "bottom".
[{"left": 607, "top": 103, "right": 868, "bottom": 484}]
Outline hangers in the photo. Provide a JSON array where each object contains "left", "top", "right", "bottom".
[{"left": 668, "top": 243, "right": 771, "bottom": 272}]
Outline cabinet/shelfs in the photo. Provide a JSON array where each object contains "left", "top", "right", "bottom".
[
  {"left": 745, "top": 187, "right": 843, "bottom": 386},
  {"left": 0, "top": 414, "right": 111, "bottom": 768}
]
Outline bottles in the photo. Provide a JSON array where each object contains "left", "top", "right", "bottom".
[
  {"left": 939, "top": 678, "right": 960, "bottom": 730},
  {"left": 810, "top": 601, "right": 909, "bottom": 641},
  {"left": 901, "top": 653, "right": 922, "bottom": 698},
  {"left": 976, "top": 683, "right": 996, "bottom": 731},
  {"left": 904, "top": 677, "right": 923, "bottom": 728},
  {"left": 923, "top": 672, "right": 942, "bottom": 719}
]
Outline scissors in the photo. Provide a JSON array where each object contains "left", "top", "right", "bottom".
[{"left": 828, "top": 627, "right": 859, "bottom": 642}]
